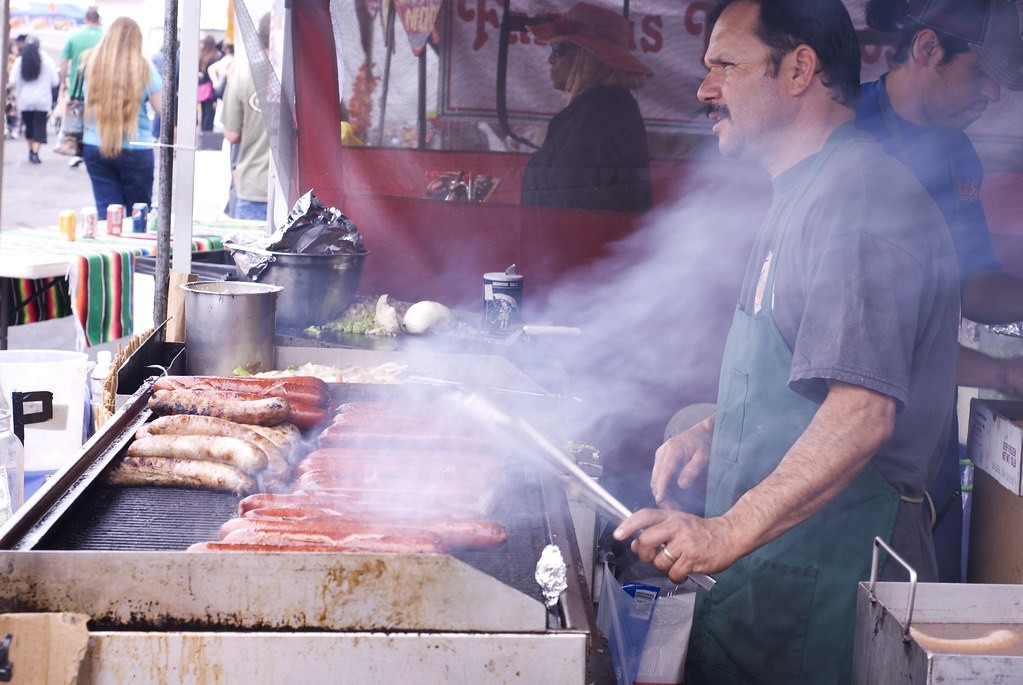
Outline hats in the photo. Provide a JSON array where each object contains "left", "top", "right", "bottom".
[
  {"left": 905, "top": 0, "right": 1023, "bottom": 91},
  {"left": 525, "top": 3, "right": 653, "bottom": 89}
]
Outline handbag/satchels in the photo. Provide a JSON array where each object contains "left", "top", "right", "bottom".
[
  {"left": 64, "top": 66, "right": 84, "bottom": 139},
  {"left": 196, "top": 81, "right": 213, "bottom": 102},
  {"left": 598, "top": 552, "right": 697, "bottom": 685}
]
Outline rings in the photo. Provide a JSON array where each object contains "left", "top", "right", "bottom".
[{"left": 663, "top": 547, "right": 676, "bottom": 562}]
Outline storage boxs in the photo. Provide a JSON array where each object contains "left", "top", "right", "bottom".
[{"left": 966, "top": 398, "right": 1023, "bottom": 584}]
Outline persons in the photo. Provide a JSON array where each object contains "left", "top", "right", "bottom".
[
  {"left": 520, "top": 2, "right": 653, "bottom": 214},
  {"left": 2, "top": 7, "right": 370, "bottom": 222},
  {"left": 614, "top": 0, "right": 1023, "bottom": 685}
]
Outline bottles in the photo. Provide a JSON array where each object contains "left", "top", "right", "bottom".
[
  {"left": 91, "top": 351, "right": 113, "bottom": 434},
  {"left": 0, "top": 408, "right": 26, "bottom": 513}
]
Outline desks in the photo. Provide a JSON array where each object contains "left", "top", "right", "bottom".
[{"left": 0, "top": 218, "right": 266, "bottom": 349}]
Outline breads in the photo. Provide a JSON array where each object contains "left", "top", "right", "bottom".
[
  {"left": 376, "top": 292, "right": 401, "bottom": 333},
  {"left": 403, "top": 300, "right": 451, "bottom": 334}
]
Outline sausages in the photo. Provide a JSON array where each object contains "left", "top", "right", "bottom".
[{"left": 103, "top": 374, "right": 510, "bottom": 554}]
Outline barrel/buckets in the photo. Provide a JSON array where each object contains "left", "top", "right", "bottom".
[
  {"left": 180, "top": 280, "right": 284, "bottom": 378},
  {"left": 222, "top": 244, "right": 375, "bottom": 338}
]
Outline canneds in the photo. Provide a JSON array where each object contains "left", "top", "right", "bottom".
[
  {"left": 150, "top": 207, "right": 158, "bottom": 231},
  {"left": 58, "top": 209, "right": 76, "bottom": 240},
  {"left": 483, "top": 265, "right": 524, "bottom": 339},
  {"left": 132, "top": 202, "right": 148, "bottom": 233},
  {"left": 107, "top": 204, "right": 123, "bottom": 236},
  {"left": 80, "top": 207, "right": 96, "bottom": 239}
]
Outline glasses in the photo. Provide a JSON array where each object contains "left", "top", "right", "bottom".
[{"left": 549, "top": 40, "right": 579, "bottom": 57}]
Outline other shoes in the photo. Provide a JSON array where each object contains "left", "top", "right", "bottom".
[{"left": 30, "top": 151, "right": 40, "bottom": 164}]
